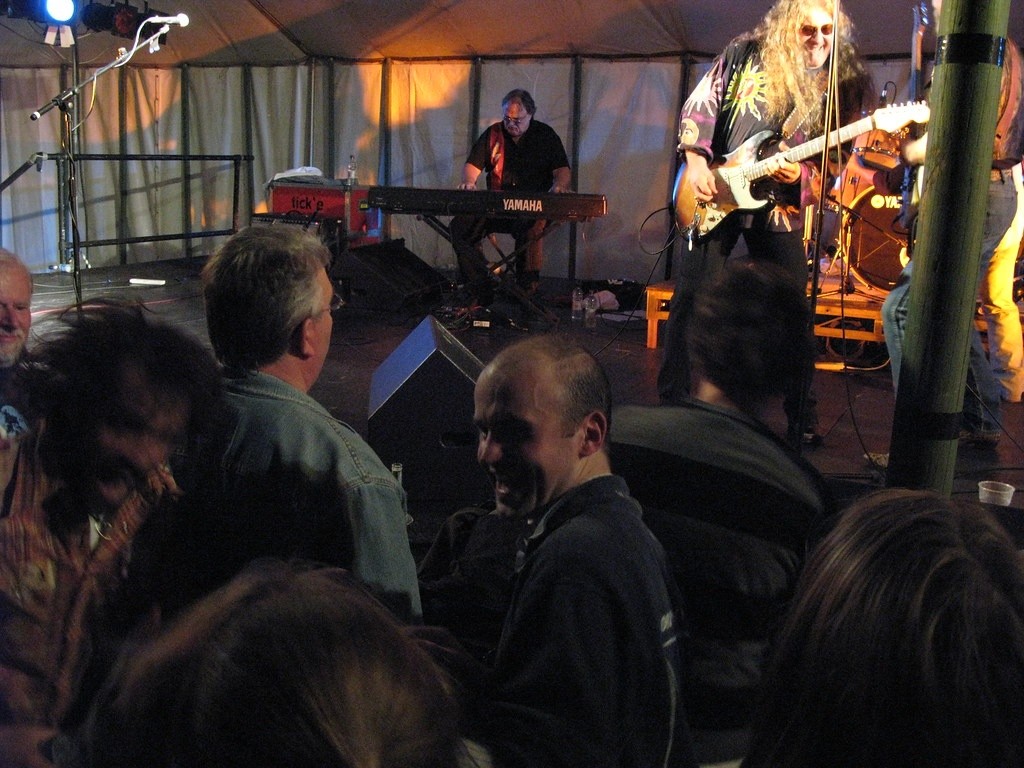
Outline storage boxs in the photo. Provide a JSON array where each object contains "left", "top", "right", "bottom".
[{"left": 267, "top": 183, "right": 384, "bottom": 248}]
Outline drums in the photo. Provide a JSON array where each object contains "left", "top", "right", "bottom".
[
  {"left": 852, "top": 130, "right": 903, "bottom": 173},
  {"left": 840, "top": 184, "right": 913, "bottom": 293},
  {"left": 820, "top": 140, "right": 874, "bottom": 214}
]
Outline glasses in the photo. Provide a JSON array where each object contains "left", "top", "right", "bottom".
[
  {"left": 501, "top": 110, "right": 532, "bottom": 123},
  {"left": 799, "top": 24, "right": 833, "bottom": 36},
  {"left": 295, "top": 293, "right": 345, "bottom": 339}
]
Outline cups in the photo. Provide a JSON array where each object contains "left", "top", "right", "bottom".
[{"left": 977, "top": 481, "right": 1015, "bottom": 506}]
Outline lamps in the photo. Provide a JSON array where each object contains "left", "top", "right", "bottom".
[{"left": 0, "top": 0, "right": 168, "bottom": 44}]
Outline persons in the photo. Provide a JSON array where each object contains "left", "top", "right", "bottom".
[
  {"left": 471, "top": 336, "right": 685, "bottom": 768},
  {"left": 0, "top": 291, "right": 247, "bottom": 768},
  {"left": 737, "top": 485, "right": 1024, "bottom": 767},
  {"left": 601, "top": 261, "right": 837, "bottom": 764},
  {"left": 76, "top": 565, "right": 479, "bottom": 768},
  {"left": 0, "top": 248, "right": 41, "bottom": 416},
  {"left": 865, "top": 0, "right": 1024, "bottom": 471},
  {"left": 446, "top": 89, "right": 576, "bottom": 315},
  {"left": 128, "top": 215, "right": 423, "bottom": 628},
  {"left": 655, "top": 0, "right": 870, "bottom": 453}
]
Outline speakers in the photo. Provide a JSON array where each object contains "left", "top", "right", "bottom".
[
  {"left": 248, "top": 213, "right": 345, "bottom": 267},
  {"left": 326, "top": 239, "right": 452, "bottom": 313},
  {"left": 364, "top": 316, "right": 497, "bottom": 501}
]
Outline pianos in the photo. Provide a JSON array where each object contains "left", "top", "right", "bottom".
[{"left": 367, "top": 186, "right": 608, "bottom": 330}]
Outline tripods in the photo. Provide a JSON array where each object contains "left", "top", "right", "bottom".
[{"left": 808, "top": 195, "right": 908, "bottom": 303}]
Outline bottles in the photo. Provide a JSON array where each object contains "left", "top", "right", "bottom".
[
  {"left": 584, "top": 290, "right": 597, "bottom": 328},
  {"left": 49, "top": 263, "right": 73, "bottom": 273},
  {"left": 347, "top": 155, "right": 359, "bottom": 186},
  {"left": 572, "top": 284, "right": 583, "bottom": 319}
]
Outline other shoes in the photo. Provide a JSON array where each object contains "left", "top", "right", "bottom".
[
  {"left": 863, "top": 453, "right": 890, "bottom": 469},
  {"left": 449, "top": 285, "right": 477, "bottom": 302},
  {"left": 526, "top": 279, "right": 544, "bottom": 296},
  {"left": 957, "top": 439, "right": 998, "bottom": 449},
  {"left": 786, "top": 425, "right": 822, "bottom": 444}
]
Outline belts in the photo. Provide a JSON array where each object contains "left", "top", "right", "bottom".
[{"left": 991, "top": 169, "right": 1012, "bottom": 181}]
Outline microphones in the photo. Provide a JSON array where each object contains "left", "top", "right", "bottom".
[
  {"left": 879, "top": 85, "right": 887, "bottom": 104},
  {"left": 147, "top": 13, "right": 190, "bottom": 27}
]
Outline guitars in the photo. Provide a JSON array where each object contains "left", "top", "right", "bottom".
[{"left": 669, "top": 100, "right": 930, "bottom": 244}]
